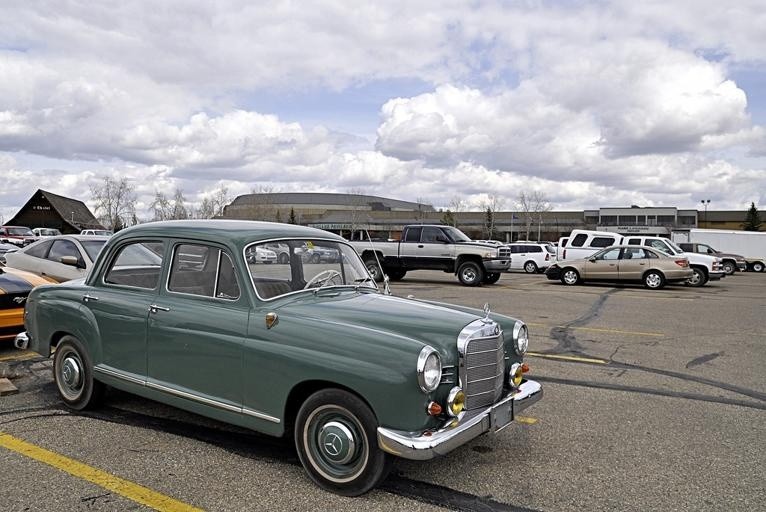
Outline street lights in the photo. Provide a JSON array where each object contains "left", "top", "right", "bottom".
[{"left": 701, "top": 199, "right": 710, "bottom": 228}]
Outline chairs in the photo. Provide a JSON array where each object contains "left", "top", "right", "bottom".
[{"left": 110, "top": 270, "right": 305, "bottom": 300}]
